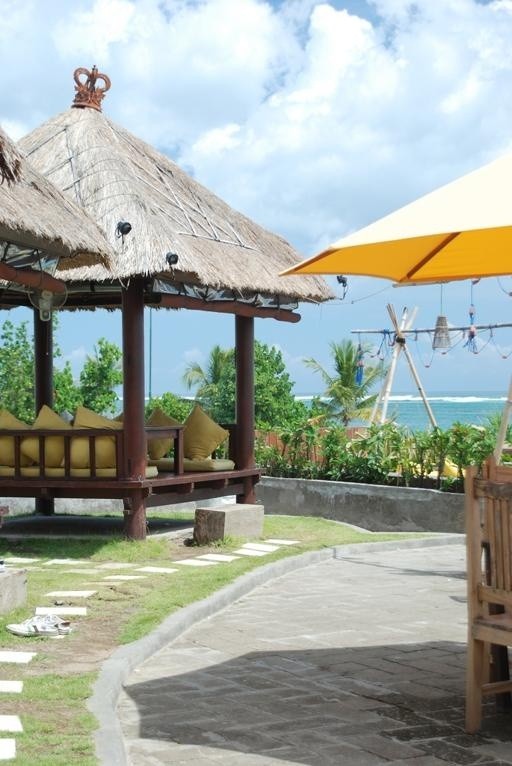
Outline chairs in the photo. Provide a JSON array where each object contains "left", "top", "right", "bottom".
[{"left": 463, "top": 465, "right": 511, "bottom": 736}]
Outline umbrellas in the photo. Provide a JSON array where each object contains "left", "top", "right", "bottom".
[{"left": 278, "top": 151, "right": 512, "bottom": 465}]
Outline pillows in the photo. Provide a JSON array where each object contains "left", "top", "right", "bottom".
[
  {"left": 146, "top": 404, "right": 231, "bottom": 460},
  {"left": 0, "top": 404, "right": 123, "bottom": 468}
]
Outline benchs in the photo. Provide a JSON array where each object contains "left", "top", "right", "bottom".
[{"left": 1, "top": 421, "right": 237, "bottom": 500}]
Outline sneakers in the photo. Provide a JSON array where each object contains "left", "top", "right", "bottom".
[
  {"left": 41, "top": 615, "right": 70, "bottom": 635},
  {"left": 6, "top": 616, "right": 59, "bottom": 636}
]
